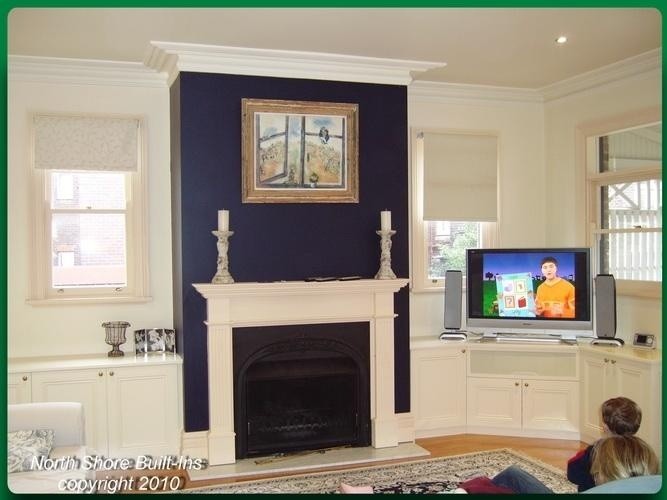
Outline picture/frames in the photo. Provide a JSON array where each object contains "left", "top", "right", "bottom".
[
  {"left": 146, "top": 328, "right": 164, "bottom": 353},
  {"left": 133, "top": 328, "right": 146, "bottom": 355},
  {"left": 240, "top": 99, "right": 361, "bottom": 206},
  {"left": 164, "top": 328, "right": 175, "bottom": 355}
]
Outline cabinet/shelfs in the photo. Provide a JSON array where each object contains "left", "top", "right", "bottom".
[
  {"left": 31, "top": 363, "right": 183, "bottom": 468},
  {"left": 469, "top": 352, "right": 581, "bottom": 439},
  {"left": 7, "top": 371, "right": 32, "bottom": 403},
  {"left": 411, "top": 347, "right": 466, "bottom": 439},
  {"left": 582, "top": 353, "right": 659, "bottom": 456}
]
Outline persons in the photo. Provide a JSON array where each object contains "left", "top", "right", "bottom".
[
  {"left": 491, "top": 436, "right": 660, "bottom": 494},
  {"left": 533, "top": 257, "right": 575, "bottom": 318},
  {"left": 568, "top": 398, "right": 643, "bottom": 493}
]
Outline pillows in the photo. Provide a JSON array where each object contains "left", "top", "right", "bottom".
[{"left": 7, "top": 429, "right": 54, "bottom": 473}]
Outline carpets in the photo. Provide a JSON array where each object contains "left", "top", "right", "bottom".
[{"left": 157, "top": 449, "right": 604, "bottom": 495}]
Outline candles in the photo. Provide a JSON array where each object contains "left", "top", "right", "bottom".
[
  {"left": 380, "top": 210, "right": 391, "bottom": 231},
  {"left": 218, "top": 209, "right": 229, "bottom": 232}
]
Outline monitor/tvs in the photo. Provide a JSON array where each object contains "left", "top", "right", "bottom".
[{"left": 465, "top": 247, "right": 593, "bottom": 342}]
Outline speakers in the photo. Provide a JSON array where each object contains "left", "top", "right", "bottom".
[
  {"left": 592, "top": 274, "right": 625, "bottom": 347},
  {"left": 438, "top": 270, "right": 468, "bottom": 340}
]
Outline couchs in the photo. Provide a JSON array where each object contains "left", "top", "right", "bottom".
[{"left": 8, "top": 401, "right": 85, "bottom": 493}]
